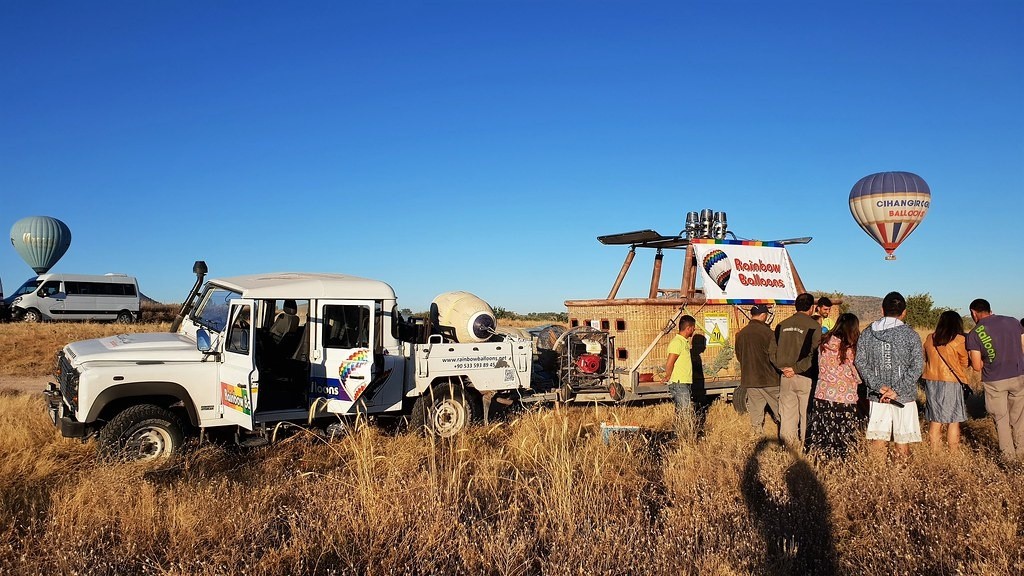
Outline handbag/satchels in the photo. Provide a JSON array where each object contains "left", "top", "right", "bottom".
[{"left": 961, "top": 382, "right": 973, "bottom": 399}]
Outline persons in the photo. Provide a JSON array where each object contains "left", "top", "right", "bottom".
[
  {"left": 810, "top": 297, "right": 836, "bottom": 333},
  {"left": 774, "top": 293, "right": 821, "bottom": 449},
  {"left": 802, "top": 313, "right": 862, "bottom": 465},
  {"left": 923, "top": 311, "right": 970, "bottom": 451},
  {"left": 656, "top": 315, "right": 696, "bottom": 416},
  {"left": 854, "top": 291, "right": 924, "bottom": 455},
  {"left": 734, "top": 304, "right": 780, "bottom": 435},
  {"left": 1020, "top": 317, "right": 1024, "bottom": 327},
  {"left": 965, "top": 298, "right": 1024, "bottom": 465}
]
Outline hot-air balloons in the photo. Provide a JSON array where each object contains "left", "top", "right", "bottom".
[
  {"left": 701, "top": 248, "right": 732, "bottom": 295},
  {"left": 849, "top": 170, "right": 932, "bottom": 260},
  {"left": 11, "top": 216, "right": 72, "bottom": 275}
]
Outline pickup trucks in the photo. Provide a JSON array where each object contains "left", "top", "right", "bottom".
[{"left": 40, "top": 259, "right": 533, "bottom": 474}]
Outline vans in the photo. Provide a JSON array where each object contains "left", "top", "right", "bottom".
[
  {"left": 6, "top": 273, "right": 142, "bottom": 324},
  {"left": 0, "top": 276, "right": 41, "bottom": 322}
]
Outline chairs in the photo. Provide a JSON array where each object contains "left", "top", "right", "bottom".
[
  {"left": 328, "top": 317, "right": 351, "bottom": 342},
  {"left": 291, "top": 316, "right": 311, "bottom": 363},
  {"left": 264, "top": 298, "right": 300, "bottom": 349}
]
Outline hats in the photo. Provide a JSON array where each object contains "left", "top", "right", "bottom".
[{"left": 750, "top": 303, "right": 774, "bottom": 315}]
